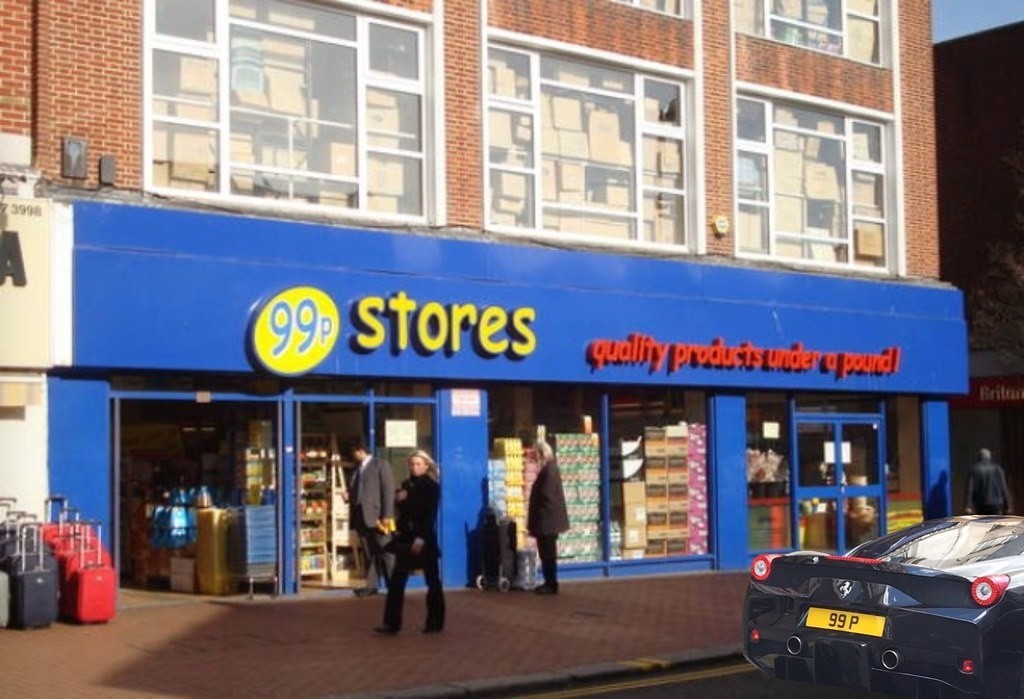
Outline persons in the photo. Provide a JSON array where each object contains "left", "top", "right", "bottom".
[
  {"left": 964, "top": 449, "right": 1012, "bottom": 515},
  {"left": 349, "top": 441, "right": 394, "bottom": 596},
  {"left": 827, "top": 465, "right": 849, "bottom": 548},
  {"left": 374, "top": 450, "right": 444, "bottom": 636},
  {"left": 527, "top": 441, "right": 570, "bottom": 594}
]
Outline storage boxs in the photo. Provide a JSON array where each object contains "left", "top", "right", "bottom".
[
  {"left": 615, "top": 421, "right": 712, "bottom": 563},
  {"left": 490, "top": 434, "right": 600, "bottom": 563},
  {"left": 156, "top": 0, "right": 886, "bottom": 273}
]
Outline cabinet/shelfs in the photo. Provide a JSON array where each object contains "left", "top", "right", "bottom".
[
  {"left": 331, "top": 435, "right": 375, "bottom": 589},
  {"left": 237, "top": 447, "right": 327, "bottom": 589}
]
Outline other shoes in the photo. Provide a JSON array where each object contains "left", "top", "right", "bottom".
[
  {"left": 374, "top": 624, "right": 402, "bottom": 634},
  {"left": 358, "top": 587, "right": 377, "bottom": 597},
  {"left": 537, "top": 581, "right": 558, "bottom": 594},
  {"left": 423, "top": 625, "right": 443, "bottom": 634}
]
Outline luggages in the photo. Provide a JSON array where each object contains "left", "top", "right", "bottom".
[{"left": 0, "top": 495, "right": 117, "bottom": 629}]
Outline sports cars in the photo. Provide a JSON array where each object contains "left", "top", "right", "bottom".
[{"left": 739, "top": 513, "right": 1023, "bottom": 699}]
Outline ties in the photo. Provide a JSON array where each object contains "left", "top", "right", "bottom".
[{"left": 352, "top": 464, "right": 360, "bottom": 503}]
